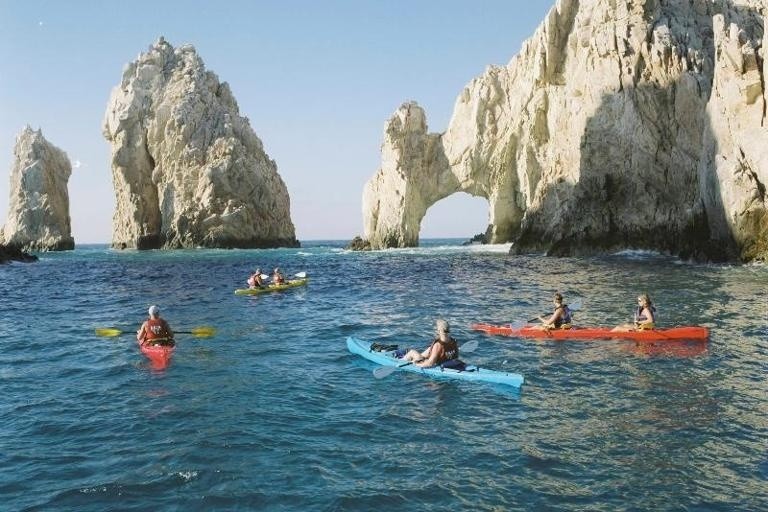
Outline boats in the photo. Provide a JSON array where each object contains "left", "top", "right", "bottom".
[
  {"left": 345, "top": 335, "right": 524, "bottom": 391},
  {"left": 233, "top": 272, "right": 306, "bottom": 296},
  {"left": 468, "top": 324, "right": 710, "bottom": 340},
  {"left": 135, "top": 337, "right": 177, "bottom": 365}
]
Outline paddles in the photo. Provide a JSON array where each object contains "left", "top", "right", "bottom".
[
  {"left": 373, "top": 340, "right": 479, "bottom": 379},
  {"left": 260, "top": 271, "right": 306, "bottom": 279},
  {"left": 95, "top": 327, "right": 213, "bottom": 337},
  {"left": 510, "top": 299, "right": 582, "bottom": 330}
]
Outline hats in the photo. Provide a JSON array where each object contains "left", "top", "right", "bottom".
[
  {"left": 437, "top": 319, "right": 449, "bottom": 335},
  {"left": 149, "top": 306, "right": 160, "bottom": 315}
]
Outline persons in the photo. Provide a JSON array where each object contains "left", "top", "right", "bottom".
[
  {"left": 270, "top": 266, "right": 285, "bottom": 285},
  {"left": 137, "top": 306, "right": 175, "bottom": 346},
  {"left": 402, "top": 319, "right": 459, "bottom": 366},
  {"left": 525, "top": 293, "right": 572, "bottom": 330},
  {"left": 247, "top": 268, "right": 264, "bottom": 289},
  {"left": 609, "top": 294, "right": 656, "bottom": 332}
]
